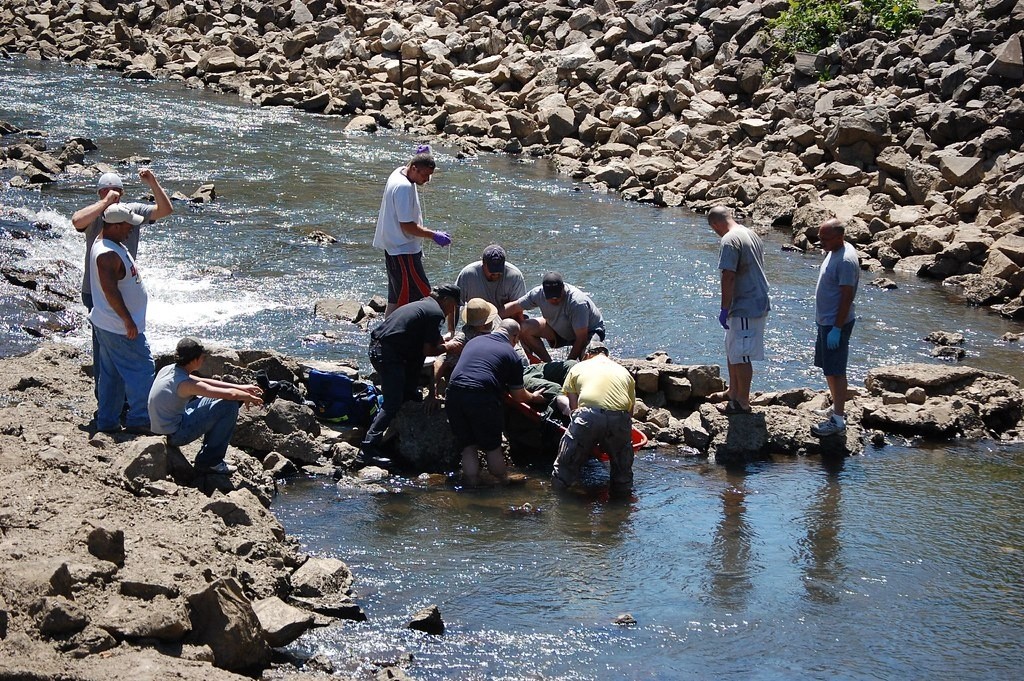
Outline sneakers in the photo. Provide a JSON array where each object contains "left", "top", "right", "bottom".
[
  {"left": 812, "top": 404, "right": 847, "bottom": 419},
  {"left": 194, "top": 460, "right": 239, "bottom": 474},
  {"left": 810, "top": 417, "right": 848, "bottom": 437}
]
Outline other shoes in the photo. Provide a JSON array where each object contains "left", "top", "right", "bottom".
[
  {"left": 97, "top": 425, "right": 127, "bottom": 432},
  {"left": 126, "top": 426, "right": 151, "bottom": 434}
]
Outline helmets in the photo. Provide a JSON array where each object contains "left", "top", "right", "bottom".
[{"left": 97, "top": 169, "right": 124, "bottom": 196}]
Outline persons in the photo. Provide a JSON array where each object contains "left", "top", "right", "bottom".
[
  {"left": 705, "top": 206, "right": 769, "bottom": 414},
  {"left": 72, "top": 169, "right": 174, "bottom": 417},
  {"left": 551, "top": 342, "right": 636, "bottom": 486},
  {"left": 810, "top": 219, "right": 860, "bottom": 438},
  {"left": 379, "top": 145, "right": 452, "bottom": 321},
  {"left": 88, "top": 202, "right": 161, "bottom": 436},
  {"left": 361, "top": 244, "right": 605, "bottom": 476},
  {"left": 148, "top": 340, "right": 265, "bottom": 474}
]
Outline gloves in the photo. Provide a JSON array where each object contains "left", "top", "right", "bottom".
[
  {"left": 719, "top": 309, "right": 730, "bottom": 330},
  {"left": 827, "top": 326, "right": 841, "bottom": 350},
  {"left": 417, "top": 145, "right": 430, "bottom": 154},
  {"left": 433, "top": 231, "right": 452, "bottom": 246}
]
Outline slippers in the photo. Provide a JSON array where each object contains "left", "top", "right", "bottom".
[
  {"left": 705, "top": 391, "right": 735, "bottom": 403},
  {"left": 717, "top": 398, "right": 752, "bottom": 412}
]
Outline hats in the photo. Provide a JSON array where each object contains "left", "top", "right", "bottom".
[
  {"left": 175, "top": 337, "right": 212, "bottom": 361},
  {"left": 460, "top": 298, "right": 497, "bottom": 326},
  {"left": 579, "top": 341, "right": 609, "bottom": 361},
  {"left": 101, "top": 202, "right": 145, "bottom": 226},
  {"left": 542, "top": 272, "right": 563, "bottom": 300},
  {"left": 483, "top": 245, "right": 507, "bottom": 273},
  {"left": 432, "top": 284, "right": 465, "bottom": 307}
]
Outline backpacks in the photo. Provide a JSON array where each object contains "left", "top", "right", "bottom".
[{"left": 308, "top": 370, "right": 380, "bottom": 426}]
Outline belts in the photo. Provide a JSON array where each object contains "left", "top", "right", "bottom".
[{"left": 600, "top": 410, "right": 626, "bottom": 415}]
